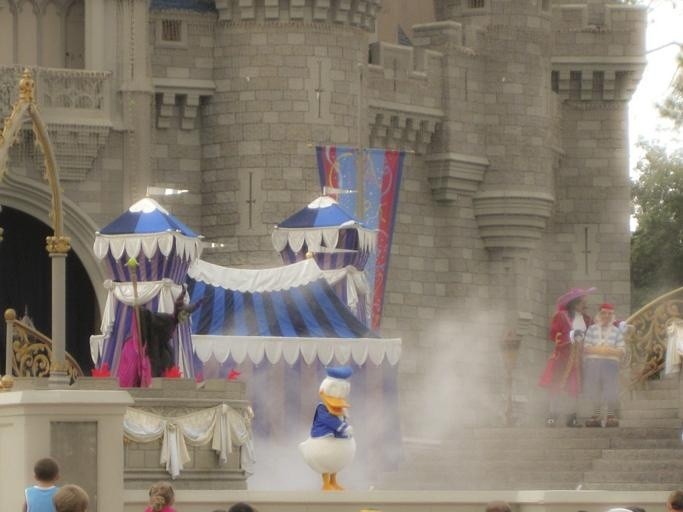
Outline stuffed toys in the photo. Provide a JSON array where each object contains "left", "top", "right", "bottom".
[{"left": 298, "top": 364, "right": 357, "bottom": 491}]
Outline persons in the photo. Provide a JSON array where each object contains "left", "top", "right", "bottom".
[
  {"left": 51, "top": 484, "right": 90, "bottom": 512},
  {"left": 486, "top": 499, "right": 511, "bottom": 512},
  {"left": 580, "top": 304, "right": 627, "bottom": 427},
  {"left": 663, "top": 302, "right": 683, "bottom": 373},
  {"left": 666, "top": 489, "right": 683, "bottom": 512},
  {"left": 536, "top": 286, "right": 635, "bottom": 428},
  {"left": 116, "top": 281, "right": 207, "bottom": 389},
  {"left": 17, "top": 456, "right": 61, "bottom": 512},
  {"left": 143, "top": 481, "right": 177, "bottom": 511}
]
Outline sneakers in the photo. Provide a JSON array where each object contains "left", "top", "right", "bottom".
[
  {"left": 607, "top": 417, "right": 619, "bottom": 426},
  {"left": 568, "top": 417, "right": 584, "bottom": 427},
  {"left": 545, "top": 417, "right": 558, "bottom": 427},
  {"left": 586, "top": 419, "right": 601, "bottom": 427}
]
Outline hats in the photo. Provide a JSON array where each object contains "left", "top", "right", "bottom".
[
  {"left": 599, "top": 303, "right": 616, "bottom": 310},
  {"left": 558, "top": 287, "right": 597, "bottom": 307}
]
